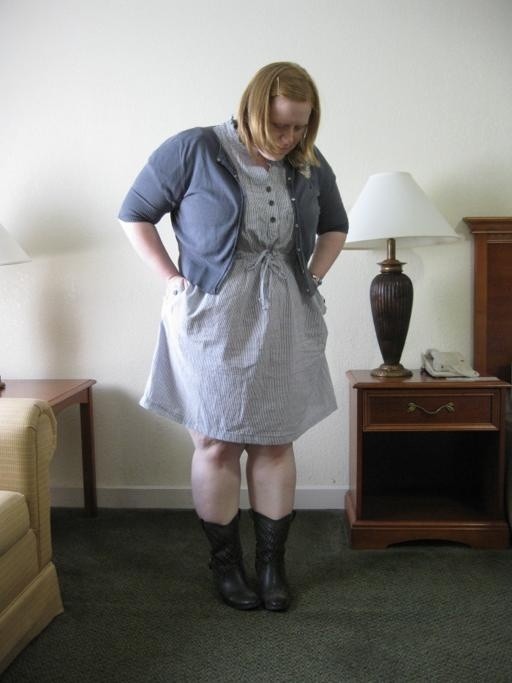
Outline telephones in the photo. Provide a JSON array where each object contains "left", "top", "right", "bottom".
[{"left": 422, "top": 349, "right": 471, "bottom": 378}]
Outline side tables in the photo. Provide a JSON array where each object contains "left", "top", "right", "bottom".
[{"left": 0, "top": 378, "right": 98, "bottom": 519}]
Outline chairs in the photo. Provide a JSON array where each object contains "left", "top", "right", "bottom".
[{"left": 0, "top": 398, "right": 64, "bottom": 676}]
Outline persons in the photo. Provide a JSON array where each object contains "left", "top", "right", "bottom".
[{"left": 118, "top": 60, "right": 350, "bottom": 613}]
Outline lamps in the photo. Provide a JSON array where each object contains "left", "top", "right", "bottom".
[
  {"left": 0, "top": 223, "right": 33, "bottom": 390},
  {"left": 314, "top": 173, "right": 461, "bottom": 377}
]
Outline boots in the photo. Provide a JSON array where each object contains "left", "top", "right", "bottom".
[
  {"left": 249, "top": 508, "right": 296, "bottom": 611},
  {"left": 196, "top": 508, "right": 261, "bottom": 610}
]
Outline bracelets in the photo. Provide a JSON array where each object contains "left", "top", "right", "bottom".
[{"left": 307, "top": 270, "right": 323, "bottom": 286}]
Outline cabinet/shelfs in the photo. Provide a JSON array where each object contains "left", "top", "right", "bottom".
[{"left": 346, "top": 369, "right": 512, "bottom": 549}]
